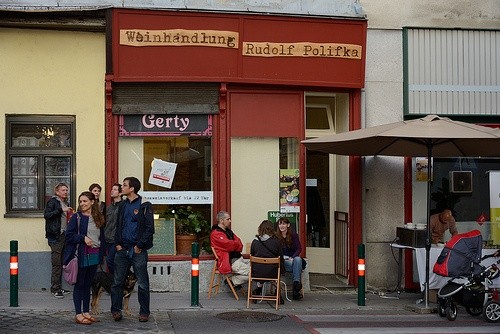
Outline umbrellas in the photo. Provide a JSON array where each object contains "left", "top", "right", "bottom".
[{"left": 302, "top": 115, "right": 500, "bottom": 310}]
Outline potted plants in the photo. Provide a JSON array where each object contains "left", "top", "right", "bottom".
[{"left": 163, "top": 205, "right": 212, "bottom": 255}]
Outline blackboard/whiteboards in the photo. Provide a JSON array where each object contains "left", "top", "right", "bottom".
[{"left": 147, "top": 218, "right": 176, "bottom": 256}]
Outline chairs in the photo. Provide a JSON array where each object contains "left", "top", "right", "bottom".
[
  {"left": 247, "top": 254, "right": 282, "bottom": 310},
  {"left": 207, "top": 245, "right": 245, "bottom": 300}
]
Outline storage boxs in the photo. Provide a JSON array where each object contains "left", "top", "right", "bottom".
[{"left": 396, "top": 227, "right": 432, "bottom": 248}]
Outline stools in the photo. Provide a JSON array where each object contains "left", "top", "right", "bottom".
[{"left": 271, "top": 270, "right": 305, "bottom": 300}]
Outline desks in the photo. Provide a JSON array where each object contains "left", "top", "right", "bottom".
[{"left": 389, "top": 243, "right": 500, "bottom": 304}]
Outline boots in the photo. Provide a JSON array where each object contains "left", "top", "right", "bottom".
[
  {"left": 274, "top": 289, "right": 284, "bottom": 304},
  {"left": 252, "top": 288, "right": 260, "bottom": 303}
]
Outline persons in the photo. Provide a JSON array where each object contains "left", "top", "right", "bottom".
[
  {"left": 211, "top": 210, "right": 252, "bottom": 297},
  {"left": 104, "top": 183, "right": 128, "bottom": 296},
  {"left": 430, "top": 209, "right": 459, "bottom": 243},
  {"left": 44, "top": 182, "right": 75, "bottom": 298},
  {"left": 66, "top": 191, "right": 104, "bottom": 324},
  {"left": 250, "top": 221, "right": 285, "bottom": 305},
  {"left": 275, "top": 217, "right": 306, "bottom": 291},
  {"left": 77, "top": 182, "right": 108, "bottom": 215},
  {"left": 110, "top": 177, "right": 154, "bottom": 321}
]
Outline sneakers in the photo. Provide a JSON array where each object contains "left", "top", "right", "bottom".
[
  {"left": 61, "top": 289, "right": 70, "bottom": 294},
  {"left": 54, "top": 292, "right": 64, "bottom": 298}
]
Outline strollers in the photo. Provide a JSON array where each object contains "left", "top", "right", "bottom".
[{"left": 433, "top": 230, "right": 500, "bottom": 323}]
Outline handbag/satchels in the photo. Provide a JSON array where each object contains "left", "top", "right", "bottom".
[
  {"left": 63, "top": 256, "right": 77, "bottom": 284},
  {"left": 280, "top": 263, "right": 286, "bottom": 273}
]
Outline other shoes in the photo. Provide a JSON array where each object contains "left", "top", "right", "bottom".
[
  {"left": 76, "top": 317, "right": 91, "bottom": 324},
  {"left": 84, "top": 316, "right": 100, "bottom": 321},
  {"left": 224, "top": 279, "right": 241, "bottom": 291},
  {"left": 113, "top": 312, "right": 122, "bottom": 320},
  {"left": 293, "top": 282, "right": 302, "bottom": 291},
  {"left": 139, "top": 314, "right": 148, "bottom": 321},
  {"left": 294, "top": 292, "right": 303, "bottom": 300}
]
